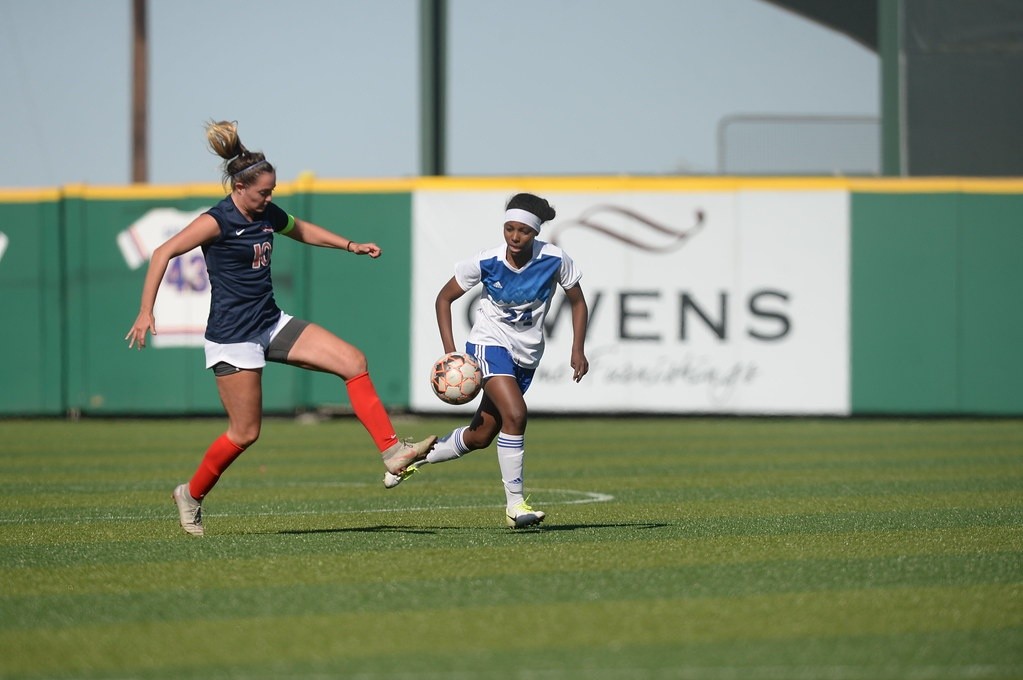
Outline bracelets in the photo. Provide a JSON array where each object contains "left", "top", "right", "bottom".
[{"left": 347, "top": 241, "right": 353, "bottom": 252}]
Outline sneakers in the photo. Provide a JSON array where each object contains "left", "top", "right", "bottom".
[
  {"left": 171, "top": 483, "right": 204, "bottom": 538},
  {"left": 381, "top": 457, "right": 428, "bottom": 489},
  {"left": 504, "top": 503, "right": 546, "bottom": 531},
  {"left": 381, "top": 434, "right": 439, "bottom": 477}
]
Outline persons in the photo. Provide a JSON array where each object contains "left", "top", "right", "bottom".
[
  {"left": 384, "top": 194, "right": 589, "bottom": 529},
  {"left": 126, "top": 120, "right": 437, "bottom": 535}
]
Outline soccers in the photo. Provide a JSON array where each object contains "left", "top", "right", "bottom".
[{"left": 430, "top": 351, "right": 483, "bottom": 406}]
